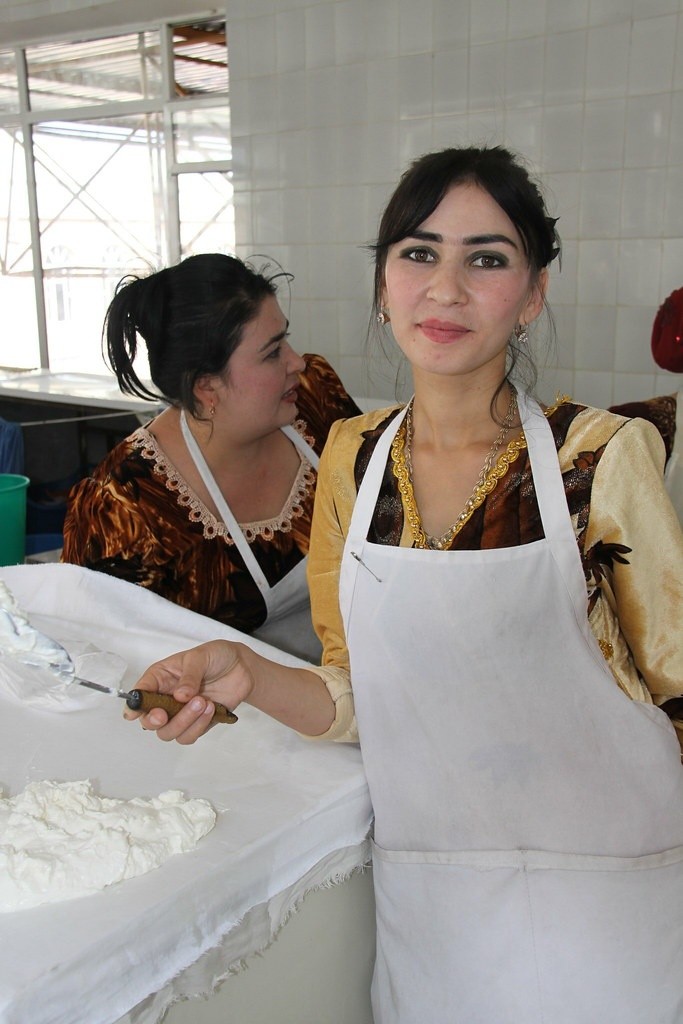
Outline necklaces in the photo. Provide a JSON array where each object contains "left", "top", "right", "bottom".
[{"left": 406, "top": 385, "right": 518, "bottom": 546}]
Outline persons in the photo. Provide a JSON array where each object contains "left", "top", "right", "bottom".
[
  {"left": 124, "top": 146, "right": 683, "bottom": 1024},
  {"left": 63, "top": 254, "right": 366, "bottom": 670}
]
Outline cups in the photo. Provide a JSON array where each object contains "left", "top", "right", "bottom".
[{"left": 0, "top": 473, "right": 30, "bottom": 568}]
